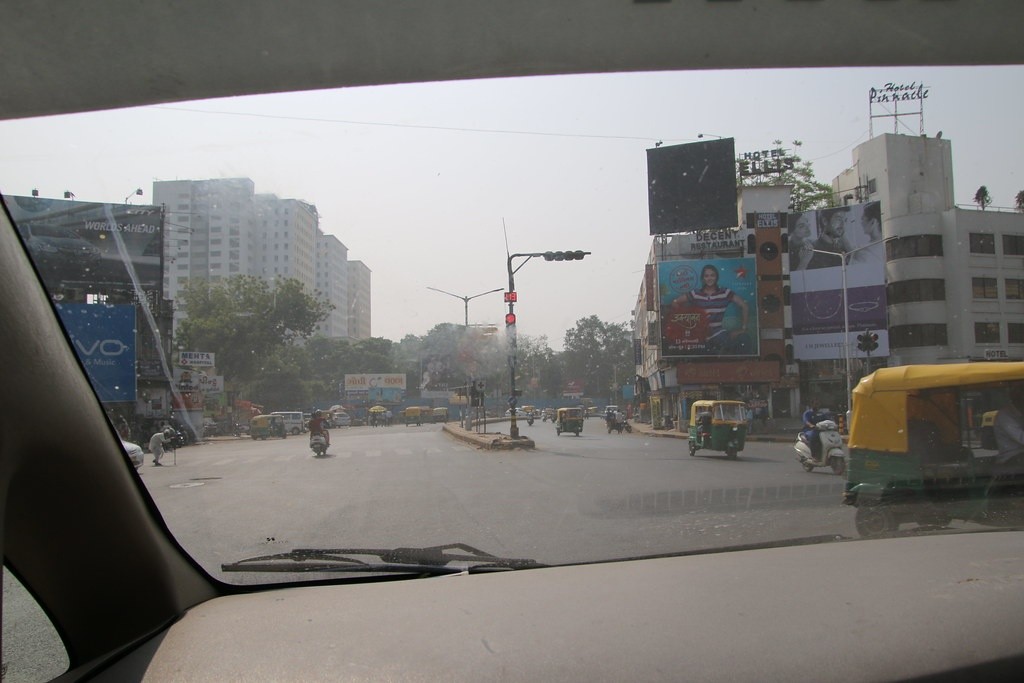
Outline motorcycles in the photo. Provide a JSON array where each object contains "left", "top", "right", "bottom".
[
  {"left": 369, "top": 406, "right": 387, "bottom": 427},
  {"left": 432, "top": 407, "right": 449, "bottom": 424},
  {"left": 555, "top": 407, "right": 584, "bottom": 437},
  {"left": 406, "top": 406, "right": 422, "bottom": 427},
  {"left": 688, "top": 399, "right": 748, "bottom": 459},
  {"left": 841, "top": 361, "right": 1024, "bottom": 538},
  {"left": 588, "top": 406, "right": 599, "bottom": 413},
  {"left": 251, "top": 414, "right": 286, "bottom": 439}
]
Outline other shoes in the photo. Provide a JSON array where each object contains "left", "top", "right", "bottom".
[{"left": 153, "top": 459, "right": 161, "bottom": 466}]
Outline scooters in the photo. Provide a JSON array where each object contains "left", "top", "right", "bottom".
[
  {"left": 606, "top": 415, "right": 632, "bottom": 434},
  {"left": 551, "top": 415, "right": 557, "bottom": 423},
  {"left": 542, "top": 411, "right": 547, "bottom": 422},
  {"left": 309, "top": 423, "right": 330, "bottom": 456},
  {"left": 527, "top": 412, "right": 535, "bottom": 426},
  {"left": 793, "top": 412, "right": 846, "bottom": 474}
]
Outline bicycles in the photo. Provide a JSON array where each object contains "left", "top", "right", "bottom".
[
  {"left": 232, "top": 419, "right": 242, "bottom": 436},
  {"left": 170, "top": 418, "right": 189, "bottom": 447}
]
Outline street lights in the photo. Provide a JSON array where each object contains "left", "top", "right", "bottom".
[
  {"left": 425, "top": 286, "right": 504, "bottom": 326},
  {"left": 604, "top": 360, "right": 628, "bottom": 405},
  {"left": 801, "top": 235, "right": 902, "bottom": 434}
]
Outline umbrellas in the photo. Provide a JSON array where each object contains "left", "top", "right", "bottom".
[{"left": 369, "top": 406, "right": 386, "bottom": 412}]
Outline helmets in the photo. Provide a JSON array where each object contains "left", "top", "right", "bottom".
[
  {"left": 316, "top": 409, "right": 321, "bottom": 415},
  {"left": 165, "top": 429, "right": 172, "bottom": 436}
]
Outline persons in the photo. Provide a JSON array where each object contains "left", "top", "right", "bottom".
[
  {"left": 696, "top": 406, "right": 714, "bottom": 432},
  {"left": 528, "top": 409, "right": 534, "bottom": 415},
  {"left": 673, "top": 265, "right": 751, "bottom": 354},
  {"left": 308, "top": 410, "right": 331, "bottom": 445},
  {"left": 606, "top": 408, "right": 625, "bottom": 434},
  {"left": 992, "top": 386, "right": 1024, "bottom": 467},
  {"left": 372, "top": 410, "right": 386, "bottom": 427},
  {"left": 788, "top": 213, "right": 850, "bottom": 271},
  {"left": 806, "top": 206, "right": 851, "bottom": 268},
  {"left": 847, "top": 201, "right": 884, "bottom": 264},
  {"left": 803, "top": 399, "right": 829, "bottom": 457},
  {"left": 148, "top": 430, "right": 169, "bottom": 466},
  {"left": 746, "top": 407, "right": 752, "bottom": 434}
]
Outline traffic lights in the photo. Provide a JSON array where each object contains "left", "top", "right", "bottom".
[
  {"left": 857, "top": 333, "right": 867, "bottom": 353},
  {"left": 867, "top": 331, "right": 879, "bottom": 351},
  {"left": 504, "top": 313, "right": 516, "bottom": 327}
]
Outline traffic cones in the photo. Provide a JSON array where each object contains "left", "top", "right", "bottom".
[{"left": 838, "top": 413, "right": 844, "bottom": 434}]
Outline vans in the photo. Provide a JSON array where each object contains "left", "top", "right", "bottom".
[{"left": 269, "top": 411, "right": 305, "bottom": 435}]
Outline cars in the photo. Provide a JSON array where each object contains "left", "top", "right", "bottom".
[
  {"left": 121, "top": 439, "right": 144, "bottom": 469},
  {"left": 303, "top": 413, "right": 312, "bottom": 431},
  {"left": 605, "top": 405, "right": 619, "bottom": 414},
  {"left": 505, "top": 405, "right": 553, "bottom": 416},
  {"left": 333, "top": 412, "right": 351, "bottom": 428}
]
